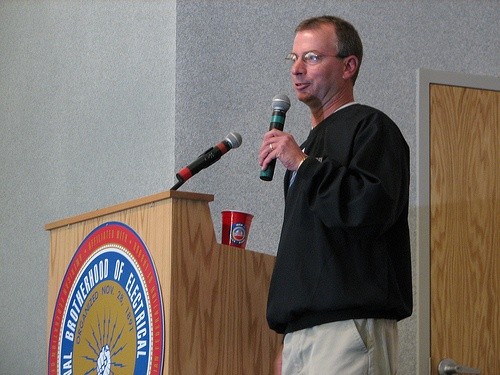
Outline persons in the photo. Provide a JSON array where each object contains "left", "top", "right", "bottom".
[{"left": 259, "top": 15, "right": 414, "bottom": 375}]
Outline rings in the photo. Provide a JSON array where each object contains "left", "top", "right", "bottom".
[{"left": 270, "top": 143, "right": 274, "bottom": 151}]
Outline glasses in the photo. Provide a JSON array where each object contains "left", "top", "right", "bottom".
[{"left": 284, "top": 51, "right": 338, "bottom": 65}]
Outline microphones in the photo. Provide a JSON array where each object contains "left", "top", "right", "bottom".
[
  {"left": 176, "top": 133, "right": 242, "bottom": 183},
  {"left": 259, "top": 95, "right": 291, "bottom": 181}
]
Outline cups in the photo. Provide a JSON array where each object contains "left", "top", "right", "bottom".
[{"left": 221, "top": 210, "right": 254, "bottom": 249}]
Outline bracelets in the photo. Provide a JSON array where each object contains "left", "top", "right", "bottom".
[{"left": 296, "top": 156, "right": 309, "bottom": 172}]
